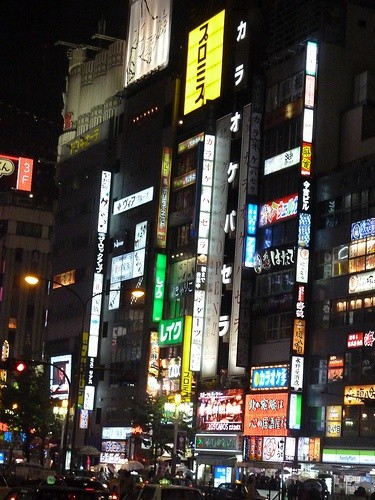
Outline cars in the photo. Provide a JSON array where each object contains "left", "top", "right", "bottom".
[{"left": 0, "top": 459, "right": 267, "bottom": 500}]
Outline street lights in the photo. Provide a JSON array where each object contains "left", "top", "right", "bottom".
[{"left": 13, "top": 272, "right": 145, "bottom": 486}]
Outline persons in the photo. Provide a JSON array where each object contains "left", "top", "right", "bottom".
[{"left": 0, "top": 460, "right": 374, "bottom": 500}]
